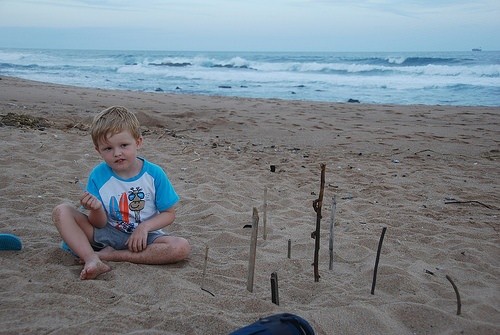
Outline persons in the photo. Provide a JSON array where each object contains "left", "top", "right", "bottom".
[{"left": 53, "top": 106, "right": 190, "bottom": 280}]
